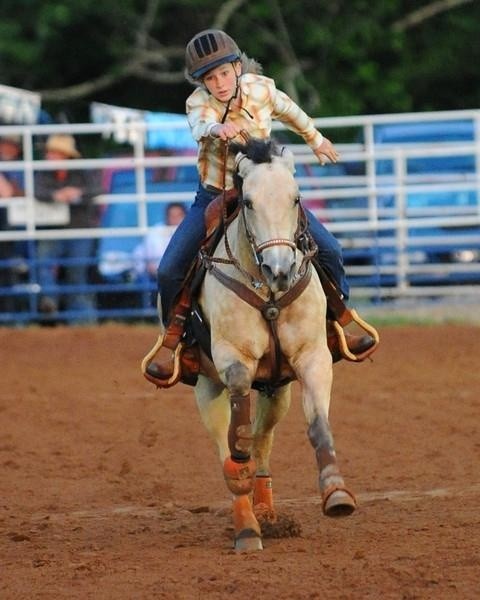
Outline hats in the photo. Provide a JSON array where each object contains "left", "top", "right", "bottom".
[{"left": 36, "top": 135, "right": 80, "bottom": 157}]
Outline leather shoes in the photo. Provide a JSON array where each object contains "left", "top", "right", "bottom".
[
  {"left": 148, "top": 357, "right": 198, "bottom": 386},
  {"left": 331, "top": 332, "right": 374, "bottom": 363}
]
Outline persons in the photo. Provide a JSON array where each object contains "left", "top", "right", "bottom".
[
  {"left": 0, "top": 131, "right": 39, "bottom": 315},
  {"left": 147, "top": 203, "right": 189, "bottom": 317},
  {"left": 147, "top": 29, "right": 374, "bottom": 380},
  {"left": 33, "top": 134, "right": 101, "bottom": 325}
]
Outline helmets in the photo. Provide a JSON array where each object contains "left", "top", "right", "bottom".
[{"left": 186, "top": 29, "right": 241, "bottom": 79}]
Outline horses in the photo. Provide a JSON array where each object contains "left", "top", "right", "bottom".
[{"left": 157, "top": 138, "right": 358, "bottom": 556}]
